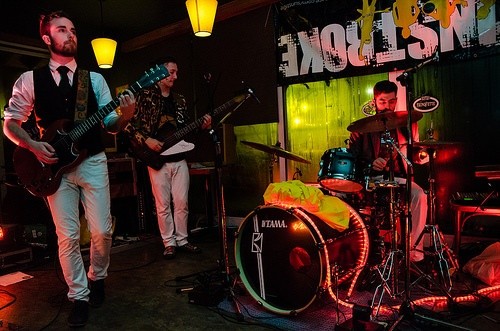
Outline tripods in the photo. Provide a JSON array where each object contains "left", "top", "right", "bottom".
[{"left": 367, "top": 118, "right": 475, "bottom": 331}]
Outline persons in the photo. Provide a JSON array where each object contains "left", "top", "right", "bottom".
[
  {"left": 3, "top": 11, "right": 136, "bottom": 329},
  {"left": 123, "top": 59, "right": 213, "bottom": 259},
  {"left": 348, "top": 80, "right": 437, "bottom": 270}
]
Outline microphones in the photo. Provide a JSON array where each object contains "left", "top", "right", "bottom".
[
  {"left": 240, "top": 78, "right": 261, "bottom": 105},
  {"left": 396, "top": 66, "right": 417, "bottom": 81}
]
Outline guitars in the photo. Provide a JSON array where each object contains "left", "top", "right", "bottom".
[
  {"left": 133, "top": 94, "right": 246, "bottom": 163},
  {"left": 11, "top": 63, "right": 170, "bottom": 198}
]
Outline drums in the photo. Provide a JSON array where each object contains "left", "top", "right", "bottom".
[
  {"left": 359, "top": 182, "right": 408, "bottom": 218},
  {"left": 232, "top": 194, "right": 370, "bottom": 313},
  {"left": 317, "top": 146, "right": 370, "bottom": 192}
]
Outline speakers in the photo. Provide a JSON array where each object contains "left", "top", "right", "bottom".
[
  {"left": 168, "top": 167, "right": 217, "bottom": 232},
  {"left": 107, "top": 157, "right": 142, "bottom": 239}
]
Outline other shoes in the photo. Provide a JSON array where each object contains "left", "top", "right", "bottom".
[
  {"left": 162, "top": 246, "right": 176, "bottom": 260},
  {"left": 410, "top": 261, "right": 434, "bottom": 288},
  {"left": 88, "top": 279, "right": 106, "bottom": 308},
  {"left": 67, "top": 300, "right": 90, "bottom": 326},
  {"left": 177, "top": 243, "right": 201, "bottom": 255}
]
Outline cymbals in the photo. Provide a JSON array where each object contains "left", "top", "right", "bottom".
[
  {"left": 410, "top": 140, "right": 467, "bottom": 167},
  {"left": 347, "top": 109, "right": 424, "bottom": 134},
  {"left": 241, "top": 139, "right": 312, "bottom": 164}
]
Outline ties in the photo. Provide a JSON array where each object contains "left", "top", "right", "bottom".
[{"left": 56, "top": 66, "right": 72, "bottom": 99}]
